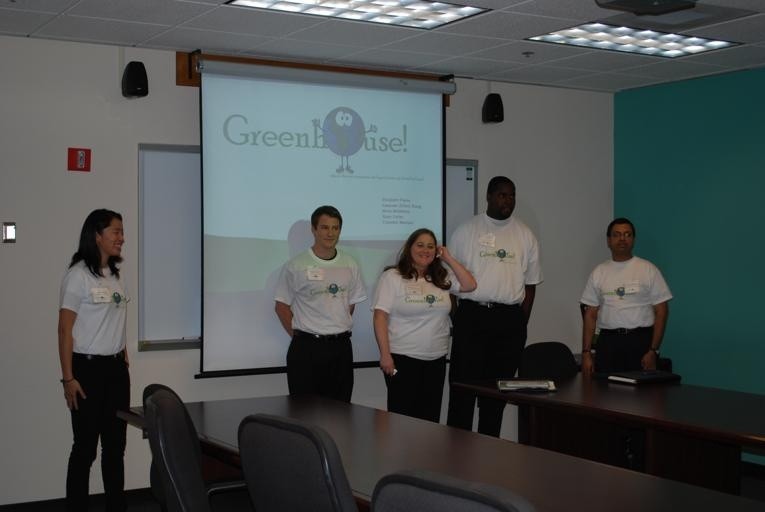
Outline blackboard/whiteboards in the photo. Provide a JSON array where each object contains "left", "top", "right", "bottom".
[{"left": 138, "top": 143, "right": 478, "bottom": 352}]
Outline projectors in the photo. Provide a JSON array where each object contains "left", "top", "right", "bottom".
[{"left": 595, "top": 0, "right": 697, "bottom": 16}]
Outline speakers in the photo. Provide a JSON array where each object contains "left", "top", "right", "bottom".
[
  {"left": 122, "top": 61, "right": 148, "bottom": 98},
  {"left": 482, "top": 93, "right": 504, "bottom": 123}
]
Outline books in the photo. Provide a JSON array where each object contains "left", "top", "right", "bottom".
[
  {"left": 497, "top": 378, "right": 557, "bottom": 395},
  {"left": 608, "top": 365, "right": 673, "bottom": 386}
]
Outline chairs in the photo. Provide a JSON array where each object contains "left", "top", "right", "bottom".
[
  {"left": 368, "top": 471, "right": 533, "bottom": 512},
  {"left": 518, "top": 341, "right": 578, "bottom": 378},
  {"left": 239, "top": 411, "right": 356, "bottom": 511},
  {"left": 136, "top": 381, "right": 245, "bottom": 511}
]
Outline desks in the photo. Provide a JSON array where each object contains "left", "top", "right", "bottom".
[
  {"left": 487, "top": 367, "right": 765, "bottom": 500},
  {"left": 114, "top": 389, "right": 763, "bottom": 512}
]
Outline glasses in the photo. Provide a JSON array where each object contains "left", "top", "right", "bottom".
[{"left": 608, "top": 231, "right": 633, "bottom": 238}]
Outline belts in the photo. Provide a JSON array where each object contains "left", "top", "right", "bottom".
[
  {"left": 599, "top": 327, "right": 646, "bottom": 334},
  {"left": 294, "top": 327, "right": 353, "bottom": 340},
  {"left": 456, "top": 297, "right": 521, "bottom": 311},
  {"left": 71, "top": 351, "right": 130, "bottom": 360}
]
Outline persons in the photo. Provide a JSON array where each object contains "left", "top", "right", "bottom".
[
  {"left": 273, "top": 205, "right": 370, "bottom": 404},
  {"left": 369, "top": 228, "right": 478, "bottom": 425},
  {"left": 579, "top": 219, "right": 674, "bottom": 379},
  {"left": 440, "top": 174, "right": 543, "bottom": 438},
  {"left": 57, "top": 208, "right": 131, "bottom": 508}
]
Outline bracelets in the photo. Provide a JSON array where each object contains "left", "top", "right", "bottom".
[
  {"left": 58, "top": 377, "right": 75, "bottom": 384},
  {"left": 649, "top": 347, "right": 661, "bottom": 357},
  {"left": 582, "top": 348, "right": 592, "bottom": 355}
]
